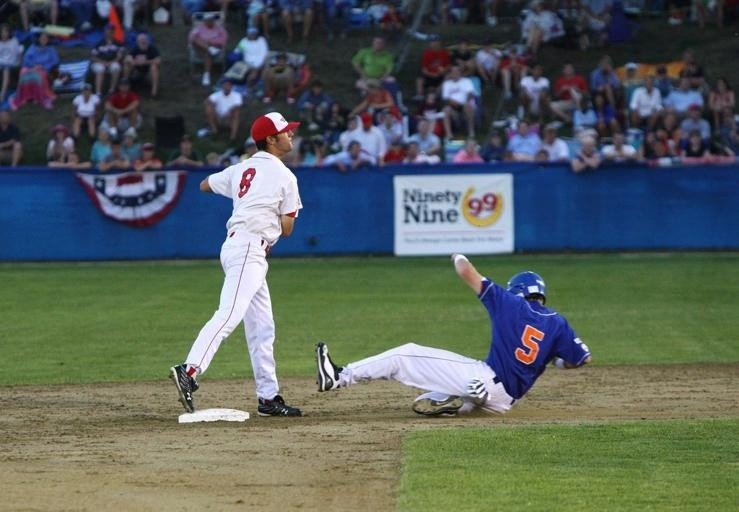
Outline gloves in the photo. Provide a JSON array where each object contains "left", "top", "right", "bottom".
[{"left": 466, "top": 378, "right": 491, "bottom": 402}]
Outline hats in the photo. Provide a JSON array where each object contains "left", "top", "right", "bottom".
[{"left": 250, "top": 111, "right": 301, "bottom": 142}]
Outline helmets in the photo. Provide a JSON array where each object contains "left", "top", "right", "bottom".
[{"left": 508, "top": 270, "right": 546, "bottom": 306}]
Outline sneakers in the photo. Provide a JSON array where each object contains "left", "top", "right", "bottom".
[
  {"left": 257, "top": 395, "right": 301, "bottom": 418},
  {"left": 412, "top": 395, "right": 462, "bottom": 416},
  {"left": 314, "top": 342, "right": 343, "bottom": 391},
  {"left": 168, "top": 364, "right": 199, "bottom": 412}
]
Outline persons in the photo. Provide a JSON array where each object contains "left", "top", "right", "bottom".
[
  {"left": 168, "top": 112, "right": 305, "bottom": 417},
  {"left": 314, "top": 251, "right": 592, "bottom": 417}
]
